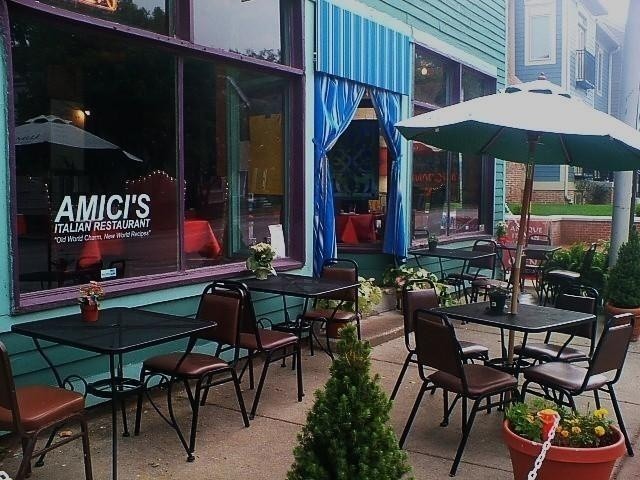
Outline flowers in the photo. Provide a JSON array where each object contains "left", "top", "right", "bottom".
[
  {"left": 504, "top": 398, "right": 610, "bottom": 446},
  {"left": 496, "top": 222, "right": 506, "bottom": 237},
  {"left": 78, "top": 282, "right": 104, "bottom": 306},
  {"left": 248, "top": 243, "right": 276, "bottom": 271}
]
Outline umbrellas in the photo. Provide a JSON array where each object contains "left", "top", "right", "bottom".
[
  {"left": 12, "top": 113, "right": 143, "bottom": 278},
  {"left": 393, "top": 70, "right": 639, "bottom": 316}
]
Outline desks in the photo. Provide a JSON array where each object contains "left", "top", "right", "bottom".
[
  {"left": 226, "top": 273, "right": 361, "bottom": 404},
  {"left": 11, "top": 306, "right": 217, "bottom": 480},
  {"left": 429, "top": 299, "right": 595, "bottom": 416},
  {"left": 407, "top": 247, "right": 496, "bottom": 325},
  {"left": 490, "top": 241, "right": 561, "bottom": 305}
]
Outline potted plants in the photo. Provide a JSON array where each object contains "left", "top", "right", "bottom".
[
  {"left": 606, "top": 241, "right": 640, "bottom": 341},
  {"left": 385, "top": 267, "right": 458, "bottom": 313},
  {"left": 318, "top": 275, "right": 381, "bottom": 339},
  {"left": 428, "top": 234, "right": 438, "bottom": 250}
]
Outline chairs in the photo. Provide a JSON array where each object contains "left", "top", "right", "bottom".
[
  {"left": 398, "top": 308, "right": 522, "bottom": 477},
  {"left": 467, "top": 254, "right": 527, "bottom": 326},
  {"left": 134, "top": 283, "right": 249, "bottom": 462},
  {"left": 387, "top": 279, "right": 491, "bottom": 414},
  {"left": 0, "top": 340, "right": 92, "bottom": 480},
  {"left": 522, "top": 234, "right": 551, "bottom": 292},
  {"left": 521, "top": 312, "right": 634, "bottom": 458},
  {"left": 540, "top": 242, "right": 597, "bottom": 305},
  {"left": 293, "top": 258, "right": 361, "bottom": 369},
  {"left": 449, "top": 240, "right": 497, "bottom": 322},
  {"left": 516, "top": 284, "right": 601, "bottom": 412},
  {"left": 211, "top": 279, "right": 301, "bottom": 419}
]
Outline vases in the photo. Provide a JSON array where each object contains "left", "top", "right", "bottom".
[
  {"left": 504, "top": 415, "right": 627, "bottom": 480},
  {"left": 499, "top": 235, "right": 507, "bottom": 245},
  {"left": 255, "top": 266, "right": 269, "bottom": 280},
  {"left": 80, "top": 302, "right": 99, "bottom": 323},
  {"left": 416, "top": 210, "right": 440, "bottom": 229}
]
